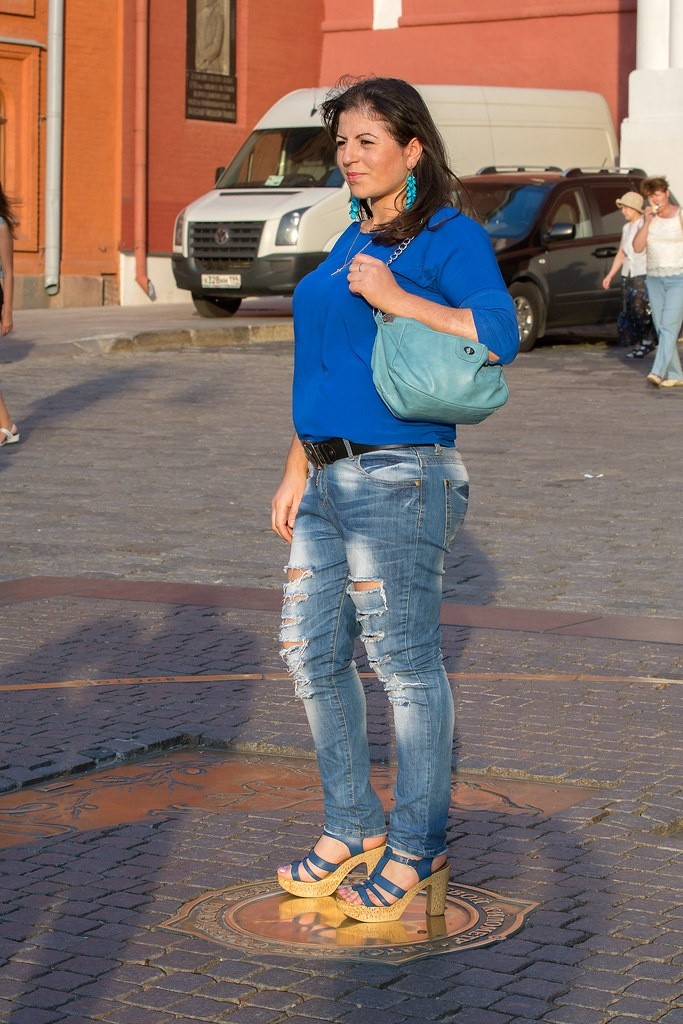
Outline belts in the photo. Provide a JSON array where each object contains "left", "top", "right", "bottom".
[{"left": 301, "top": 437, "right": 446, "bottom": 471}]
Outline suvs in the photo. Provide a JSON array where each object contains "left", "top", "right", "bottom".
[{"left": 453, "top": 165, "right": 680, "bottom": 353}]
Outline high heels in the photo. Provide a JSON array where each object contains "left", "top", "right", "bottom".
[
  {"left": 334, "top": 847, "right": 451, "bottom": 923},
  {"left": 277, "top": 827, "right": 389, "bottom": 898}
]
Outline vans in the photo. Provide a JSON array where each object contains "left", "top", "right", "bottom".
[{"left": 171, "top": 85, "right": 619, "bottom": 319}]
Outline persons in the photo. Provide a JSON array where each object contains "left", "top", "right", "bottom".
[
  {"left": 602, "top": 175, "right": 683, "bottom": 387},
  {"left": 278, "top": 897, "right": 447, "bottom": 944},
  {"left": 0, "top": 181, "right": 24, "bottom": 447},
  {"left": 271, "top": 73, "right": 519, "bottom": 923}
]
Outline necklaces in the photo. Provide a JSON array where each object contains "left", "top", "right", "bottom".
[{"left": 331, "top": 217, "right": 380, "bottom": 276}]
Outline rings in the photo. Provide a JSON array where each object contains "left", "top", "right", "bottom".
[{"left": 359, "top": 263, "right": 364, "bottom": 272}]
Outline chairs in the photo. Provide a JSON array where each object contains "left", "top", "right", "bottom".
[
  {"left": 298, "top": 158, "right": 327, "bottom": 180},
  {"left": 549, "top": 204, "right": 577, "bottom": 228}
]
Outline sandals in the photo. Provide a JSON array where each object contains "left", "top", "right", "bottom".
[
  {"left": 0, "top": 424, "right": 20, "bottom": 446},
  {"left": 626, "top": 348, "right": 644, "bottom": 358},
  {"left": 636, "top": 342, "right": 657, "bottom": 356}
]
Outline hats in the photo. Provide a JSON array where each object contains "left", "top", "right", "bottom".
[{"left": 615, "top": 191, "right": 645, "bottom": 215}]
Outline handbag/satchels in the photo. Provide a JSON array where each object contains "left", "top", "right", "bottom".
[{"left": 370, "top": 215, "right": 509, "bottom": 424}]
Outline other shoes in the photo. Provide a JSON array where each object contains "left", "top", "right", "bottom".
[
  {"left": 659, "top": 379, "right": 683, "bottom": 387},
  {"left": 646, "top": 373, "right": 663, "bottom": 386}
]
